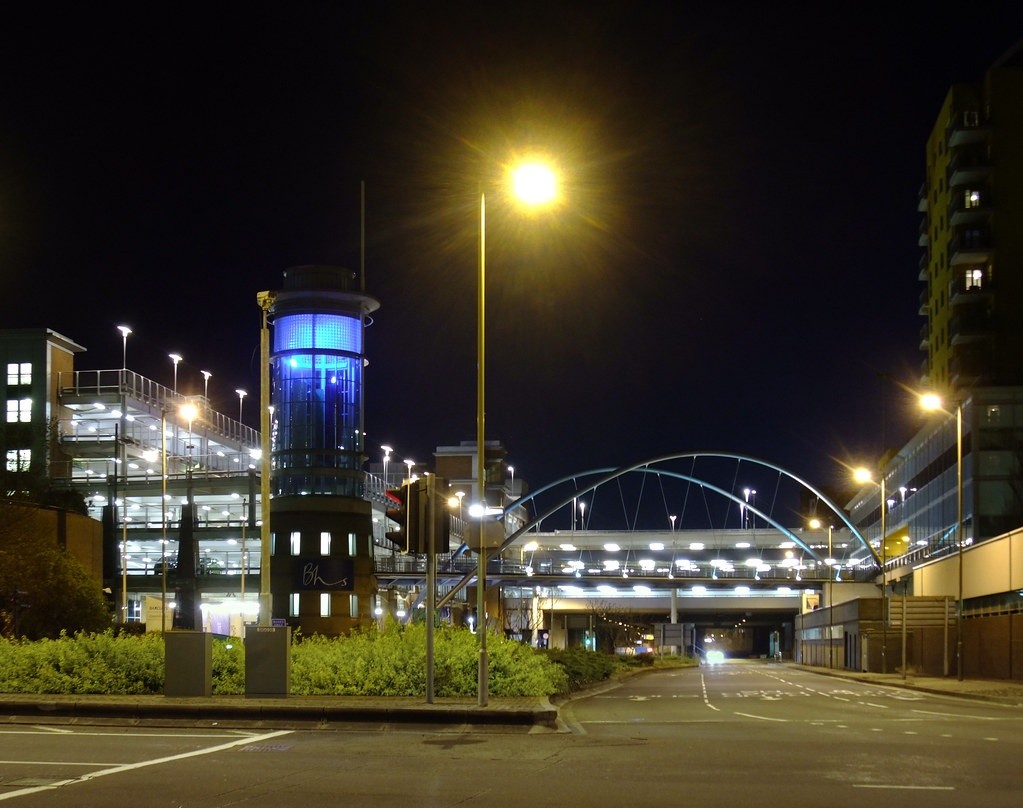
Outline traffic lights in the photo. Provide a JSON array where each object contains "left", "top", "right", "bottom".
[{"left": 386, "top": 480, "right": 420, "bottom": 555}]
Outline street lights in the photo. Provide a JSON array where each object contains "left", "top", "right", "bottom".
[
  {"left": 809, "top": 519, "right": 833, "bottom": 670},
  {"left": 853, "top": 467, "right": 887, "bottom": 672},
  {"left": 479, "top": 150, "right": 567, "bottom": 705},
  {"left": 161, "top": 401, "right": 200, "bottom": 631},
  {"left": 920, "top": 404, "right": 963, "bottom": 681}
]
